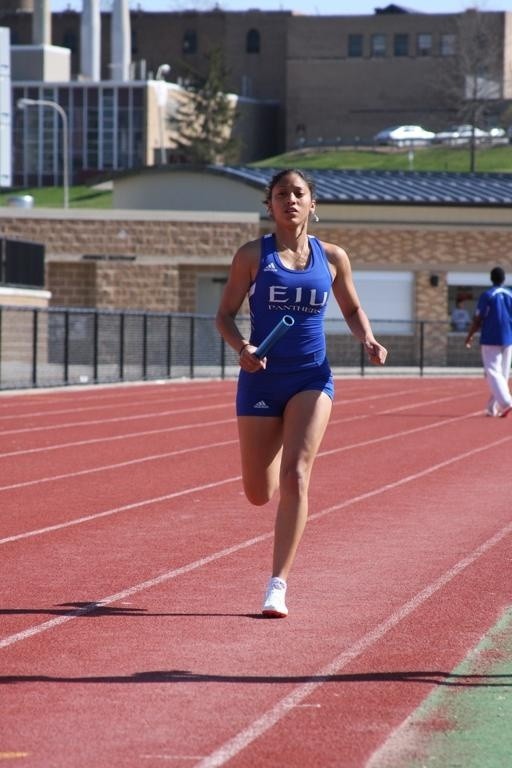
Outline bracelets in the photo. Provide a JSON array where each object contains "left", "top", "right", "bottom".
[{"left": 237, "top": 343, "right": 251, "bottom": 355}]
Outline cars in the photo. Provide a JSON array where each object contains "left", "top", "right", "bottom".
[
  {"left": 435, "top": 124, "right": 490, "bottom": 142},
  {"left": 374, "top": 124, "right": 436, "bottom": 145}
]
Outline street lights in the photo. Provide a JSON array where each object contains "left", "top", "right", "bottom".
[{"left": 16, "top": 97, "right": 69, "bottom": 208}]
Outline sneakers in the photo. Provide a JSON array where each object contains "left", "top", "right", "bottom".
[
  {"left": 262, "top": 580, "right": 288, "bottom": 618},
  {"left": 484, "top": 403, "right": 512, "bottom": 418}
]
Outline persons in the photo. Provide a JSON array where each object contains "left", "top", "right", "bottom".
[
  {"left": 214, "top": 167, "right": 388, "bottom": 615},
  {"left": 465, "top": 265, "right": 512, "bottom": 418},
  {"left": 452, "top": 301, "right": 472, "bottom": 331}
]
